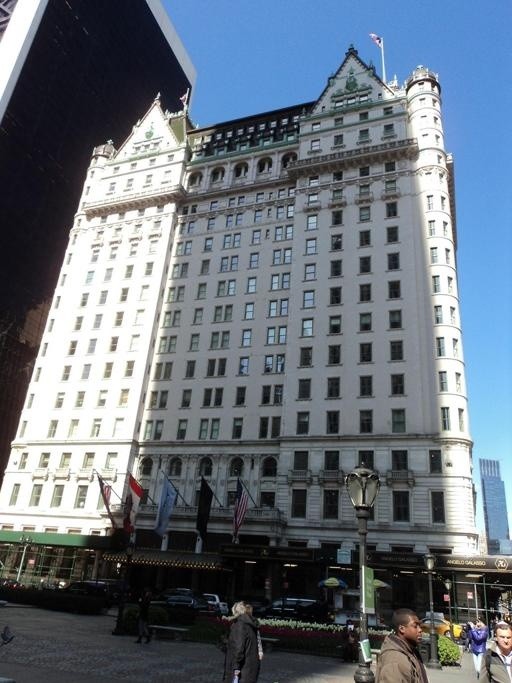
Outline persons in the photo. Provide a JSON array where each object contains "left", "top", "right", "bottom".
[
  {"left": 223, "top": 600, "right": 263, "bottom": 683},
  {"left": 133, "top": 591, "right": 153, "bottom": 644},
  {"left": 375, "top": 608, "right": 428, "bottom": 683},
  {"left": 466, "top": 616, "right": 488, "bottom": 678},
  {"left": 479, "top": 621, "right": 512, "bottom": 683}
]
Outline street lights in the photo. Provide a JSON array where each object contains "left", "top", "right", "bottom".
[
  {"left": 444, "top": 579, "right": 456, "bottom": 640},
  {"left": 16, "top": 535, "right": 32, "bottom": 582},
  {"left": 421, "top": 554, "right": 443, "bottom": 669},
  {"left": 345, "top": 459, "right": 381, "bottom": 683}
]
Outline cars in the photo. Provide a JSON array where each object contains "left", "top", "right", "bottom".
[
  {"left": 151, "top": 588, "right": 229, "bottom": 615},
  {"left": 68, "top": 580, "right": 110, "bottom": 615},
  {"left": 419, "top": 616, "right": 466, "bottom": 641}
]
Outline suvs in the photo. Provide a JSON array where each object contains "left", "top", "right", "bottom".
[{"left": 256, "top": 597, "right": 335, "bottom": 624}]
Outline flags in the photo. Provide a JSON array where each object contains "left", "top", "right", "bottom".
[
  {"left": 369, "top": 32, "right": 382, "bottom": 47},
  {"left": 121, "top": 474, "right": 143, "bottom": 533},
  {"left": 232, "top": 477, "right": 249, "bottom": 544},
  {"left": 195, "top": 476, "right": 213, "bottom": 541},
  {"left": 154, "top": 476, "right": 179, "bottom": 537},
  {"left": 98, "top": 476, "right": 116, "bottom": 528}
]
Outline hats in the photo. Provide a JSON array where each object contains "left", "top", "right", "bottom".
[{"left": 478, "top": 615, "right": 487, "bottom": 624}]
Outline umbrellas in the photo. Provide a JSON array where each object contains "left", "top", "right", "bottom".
[
  {"left": 358, "top": 579, "right": 392, "bottom": 608},
  {"left": 318, "top": 576, "right": 348, "bottom": 600}
]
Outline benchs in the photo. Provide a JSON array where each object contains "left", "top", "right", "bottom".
[
  {"left": 147, "top": 624, "right": 191, "bottom": 643},
  {"left": 261, "top": 637, "right": 281, "bottom": 652}
]
